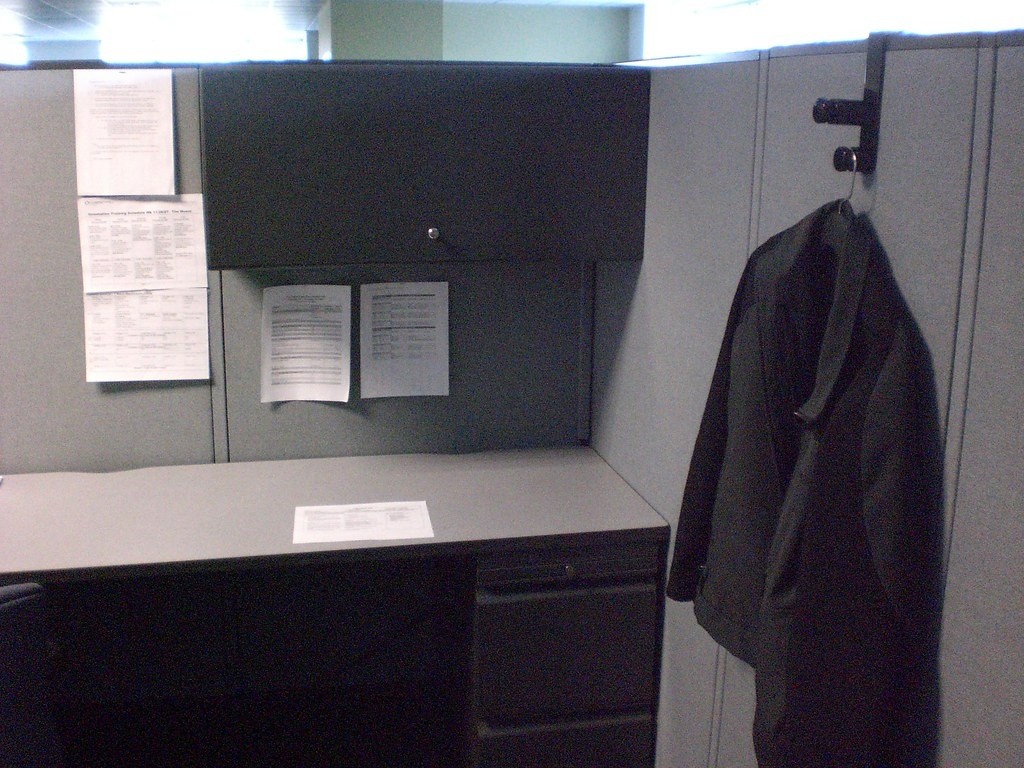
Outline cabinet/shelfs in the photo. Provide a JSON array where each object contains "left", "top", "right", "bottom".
[{"left": 195, "top": 63, "right": 652, "bottom": 270}]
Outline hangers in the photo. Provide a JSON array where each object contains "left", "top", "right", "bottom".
[{"left": 838, "top": 147, "right": 867, "bottom": 215}]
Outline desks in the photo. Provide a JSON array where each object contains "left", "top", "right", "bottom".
[{"left": 0, "top": 443, "right": 671, "bottom": 768}]
